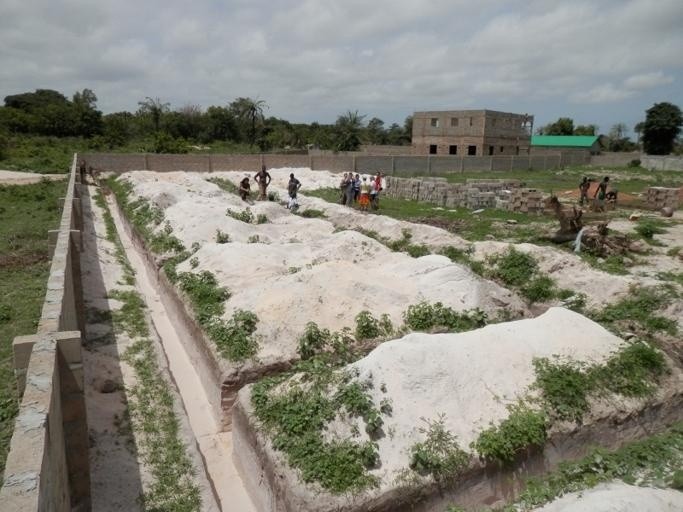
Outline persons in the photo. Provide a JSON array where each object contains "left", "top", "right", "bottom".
[
  {"left": 237, "top": 178, "right": 251, "bottom": 200},
  {"left": 285, "top": 173, "right": 300, "bottom": 197},
  {"left": 604, "top": 188, "right": 618, "bottom": 200},
  {"left": 578, "top": 176, "right": 590, "bottom": 204},
  {"left": 78, "top": 160, "right": 87, "bottom": 184},
  {"left": 592, "top": 177, "right": 609, "bottom": 201},
  {"left": 286, "top": 191, "right": 299, "bottom": 213},
  {"left": 338, "top": 171, "right": 383, "bottom": 210},
  {"left": 253, "top": 165, "right": 271, "bottom": 201}
]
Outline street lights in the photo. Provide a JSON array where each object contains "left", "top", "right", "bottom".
[{"left": 368, "top": 195, "right": 372, "bottom": 201}]
